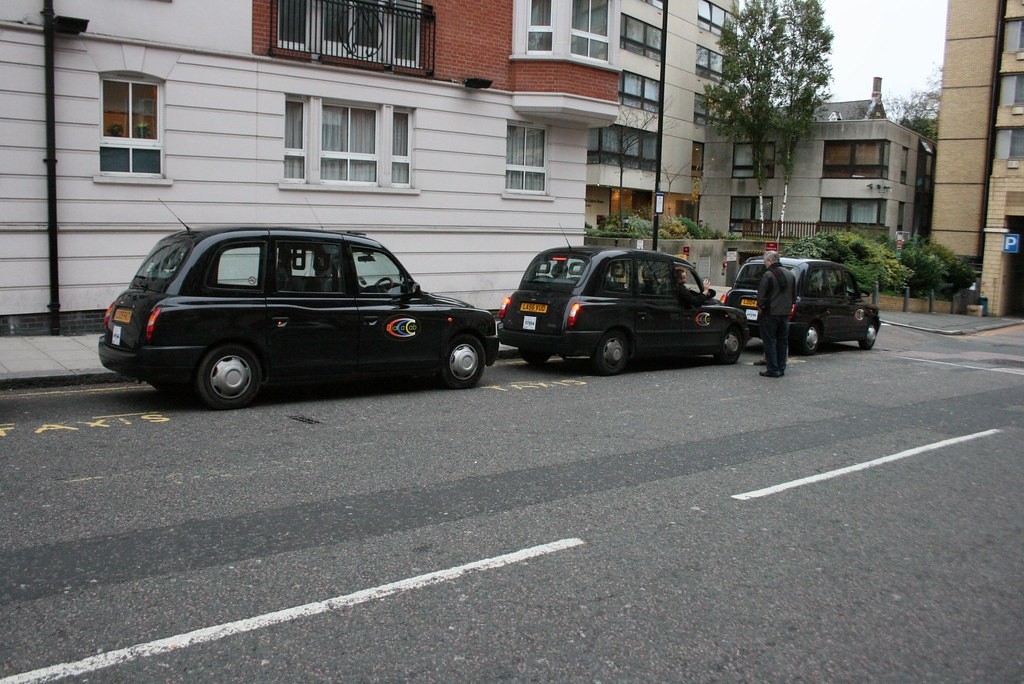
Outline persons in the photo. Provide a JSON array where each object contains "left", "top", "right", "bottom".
[
  {"left": 677, "top": 269, "right": 711, "bottom": 306},
  {"left": 753, "top": 250, "right": 797, "bottom": 377}
]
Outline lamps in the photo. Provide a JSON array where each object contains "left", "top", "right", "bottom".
[
  {"left": 54, "top": 15, "right": 88, "bottom": 34},
  {"left": 463, "top": 77, "right": 494, "bottom": 89}
]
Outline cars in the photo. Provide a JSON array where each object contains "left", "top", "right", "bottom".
[
  {"left": 718, "top": 254, "right": 880, "bottom": 357},
  {"left": 97, "top": 224, "right": 499, "bottom": 411},
  {"left": 498, "top": 244, "right": 751, "bottom": 376}
]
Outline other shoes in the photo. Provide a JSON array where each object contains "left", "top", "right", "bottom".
[
  {"left": 759, "top": 370, "right": 785, "bottom": 377},
  {"left": 753, "top": 359, "right": 767, "bottom": 366}
]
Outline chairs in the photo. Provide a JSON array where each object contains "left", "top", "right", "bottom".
[
  {"left": 568, "top": 262, "right": 584, "bottom": 281},
  {"left": 611, "top": 263, "right": 626, "bottom": 287},
  {"left": 307, "top": 262, "right": 336, "bottom": 292},
  {"left": 535, "top": 262, "right": 550, "bottom": 278}
]
